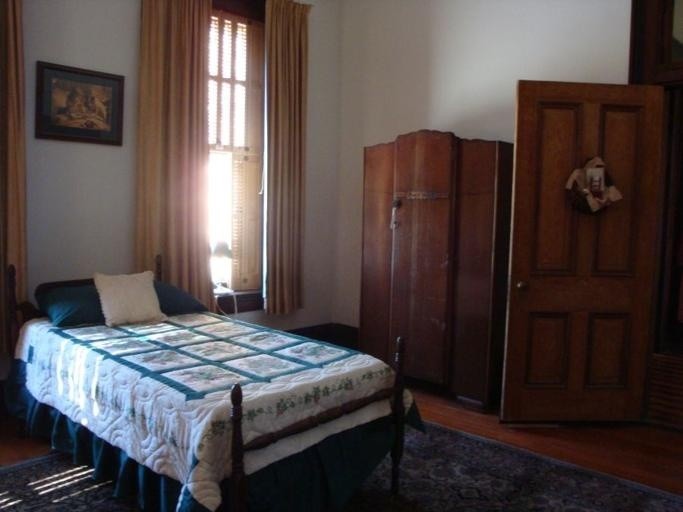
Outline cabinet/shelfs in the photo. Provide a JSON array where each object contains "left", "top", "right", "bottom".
[{"left": 360, "top": 129, "right": 503, "bottom": 414}]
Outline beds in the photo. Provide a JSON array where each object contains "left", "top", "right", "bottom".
[{"left": 6, "top": 255, "right": 406, "bottom": 512}]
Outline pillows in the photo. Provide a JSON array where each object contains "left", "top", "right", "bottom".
[
  {"left": 93, "top": 270, "right": 168, "bottom": 328},
  {"left": 35, "top": 270, "right": 208, "bottom": 329}
]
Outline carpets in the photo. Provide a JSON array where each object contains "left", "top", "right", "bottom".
[{"left": 0, "top": 419, "right": 683, "bottom": 512}]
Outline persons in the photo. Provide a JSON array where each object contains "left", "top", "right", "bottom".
[{"left": 58, "top": 87, "right": 107, "bottom": 121}]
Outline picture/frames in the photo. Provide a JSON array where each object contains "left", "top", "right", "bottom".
[{"left": 34, "top": 58, "right": 125, "bottom": 147}]
